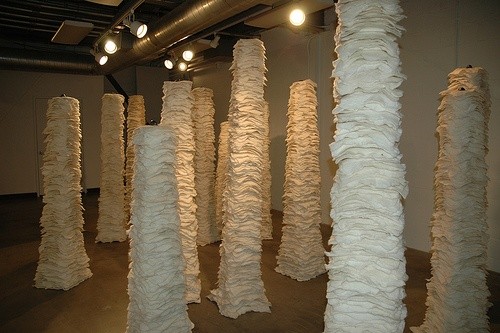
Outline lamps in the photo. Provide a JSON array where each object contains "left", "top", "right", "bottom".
[
  {"left": 163, "top": 34, "right": 220, "bottom": 71},
  {"left": 89, "top": 13, "right": 148, "bottom": 66}
]
